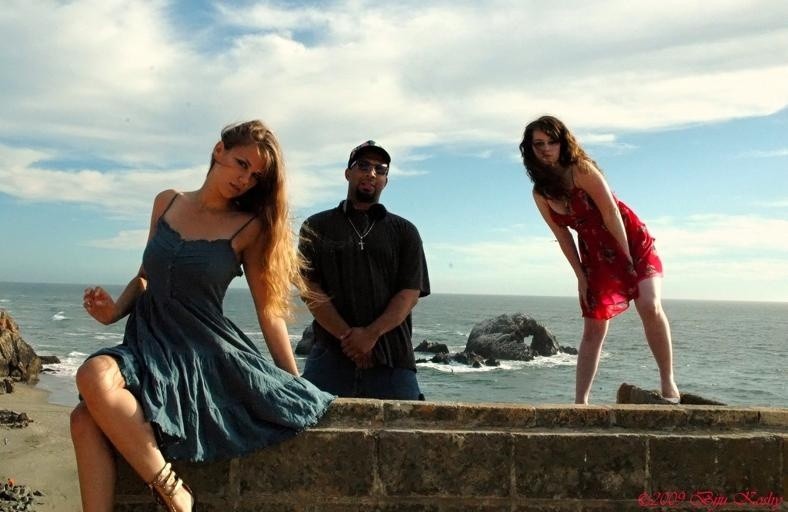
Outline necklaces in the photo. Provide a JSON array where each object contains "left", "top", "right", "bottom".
[{"left": 347, "top": 212, "right": 374, "bottom": 251}]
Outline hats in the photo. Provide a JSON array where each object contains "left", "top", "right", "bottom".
[{"left": 348, "top": 140, "right": 391, "bottom": 164}]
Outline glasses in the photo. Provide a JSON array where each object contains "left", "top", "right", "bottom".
[{"left": 351, "top": 160, "right": 389, "bottom": 176}]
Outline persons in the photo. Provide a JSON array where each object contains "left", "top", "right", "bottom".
[
  {"left": 519, "top": 116, "right": 682, "bottom": 405},
  {"left": 70, "top": 121, "right": 337, "bottom": 512},
  {"left": 296, "top": 140, "right": 431, "bottom": 401}
]
